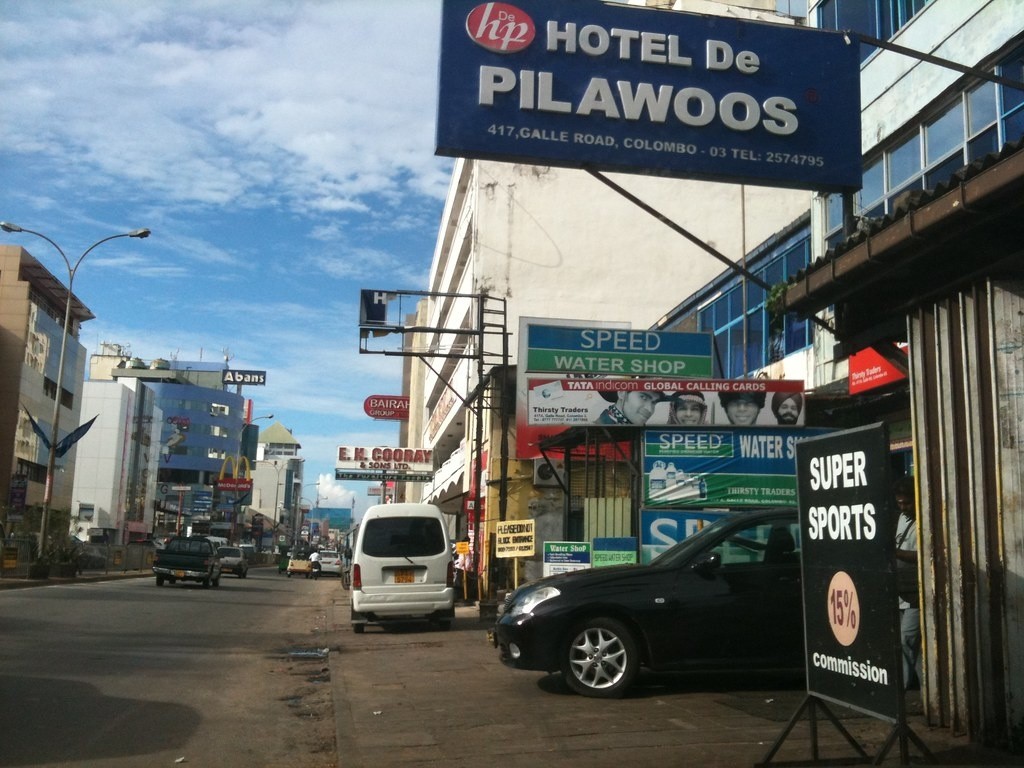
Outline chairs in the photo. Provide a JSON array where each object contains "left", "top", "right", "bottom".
[{"left": 764, "top": 522, "right": 795, "bottom": 561}]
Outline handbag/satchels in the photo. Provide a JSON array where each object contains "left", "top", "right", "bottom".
[{"left": 896, "top": 561, "right": 918, "bottom": 603}]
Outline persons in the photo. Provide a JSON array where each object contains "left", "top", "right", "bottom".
[
  {"left": 344, "top": 545, "right": 352, "bottom": 565},
  {"left": 592, "top": 380, "right": 802, "bottom": 425},
  {"left": 895, "top": 475, "right": 923, "bottom": 704},
  {"left": 308, "top": 548, "right": 322, "bottom": 579},
  {"left": 453, "top": 536, "right": 472, "bottom": 589}
]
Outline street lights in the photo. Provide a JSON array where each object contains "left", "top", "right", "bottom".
[
  {"left": 278, "top": 482, "right": 320, "bottom": 545},
  {"left": 234, "top": 414, "right": 274, "bottom": 540},
  {"left": 299, "top": 496, "right": 328, "bottom": 547},
  {"left": 252, "top": 459, "right": 305, "bottom": 544},
  {"left": 1, "top": 221, "right": 151, "bottom": 560}
]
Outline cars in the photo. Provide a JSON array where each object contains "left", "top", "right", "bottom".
[
  {"left": 217, "top": 547, "right": 249, "bottom": 578},
  {"left": 317, "top": 550, "right": 345, "bottom": 577},
  {"left": 493, "top": 506, "right": 805, "bottom": 698},
  {"left": 287, "top": 545, "right": 313, "bottom": 579}
]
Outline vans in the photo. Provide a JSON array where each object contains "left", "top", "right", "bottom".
[{"left": 350, "top": 503, "right": 456, "bottom": 633}]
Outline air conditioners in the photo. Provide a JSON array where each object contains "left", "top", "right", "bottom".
[{"left": 533, "top": 458, "right": 565, "bottom": 485}]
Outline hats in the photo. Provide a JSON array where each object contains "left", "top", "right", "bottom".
[{"left": 597, "top": 390, "right": 672, "bottom": 402}]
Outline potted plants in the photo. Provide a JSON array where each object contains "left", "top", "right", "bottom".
[{"left": 763, "top": 282, "right": 786, "bottom": 314}]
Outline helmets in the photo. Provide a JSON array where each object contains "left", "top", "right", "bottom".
[{"left": 718, "top": 391, "right": 767, "bottom": 408}]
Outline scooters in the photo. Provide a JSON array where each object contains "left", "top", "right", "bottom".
[{"left": 309, "top": 559, "right": 322, "bottom": 579}]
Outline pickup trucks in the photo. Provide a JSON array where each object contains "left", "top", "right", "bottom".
[{"left": 152, "top": 537, "right": 222, "bottom": 589}]
